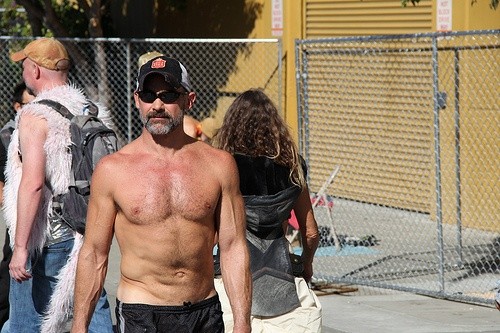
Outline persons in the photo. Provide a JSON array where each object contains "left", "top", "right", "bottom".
[
  {"left": 213, "top": 90, "right": 322, "bottom": 333},
  {"left": 70, "top": 56, "right": 252, "bottom": 333},
  {"left": 1, "top": 38, "right": 114, "bottom": 333},
  {"left": 0, "top": 82, "right": 36, "bottom": 329}
]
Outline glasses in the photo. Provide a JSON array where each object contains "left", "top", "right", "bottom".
[{"left": 134, "top": 90, "right": 188, "bottom": 104}]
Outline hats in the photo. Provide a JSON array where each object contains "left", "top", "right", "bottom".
[
  {"left": 139, "top": 51, "right": 165, "bottom": 69},
  {"left": 135, "top": 56, "right": 191, "bottom": 93},
  {"left": 11, "top": 37, "right": 70, "bottom": 71}
]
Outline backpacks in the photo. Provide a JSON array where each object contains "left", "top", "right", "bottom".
[{"left": 17, "top": 92, "right": 119, "bottom": 236}]
